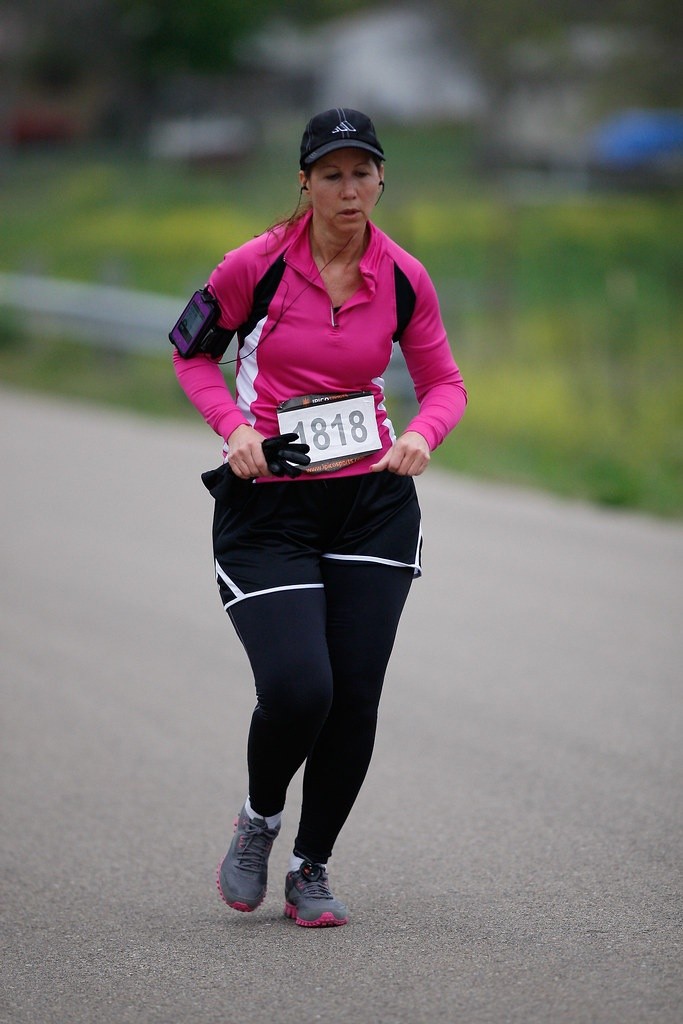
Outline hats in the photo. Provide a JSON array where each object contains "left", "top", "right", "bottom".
[{"left": 301, "top": 109, "right": 386, "bottom": 165}]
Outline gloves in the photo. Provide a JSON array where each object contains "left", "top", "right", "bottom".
[{"left": 202, "top": 433, "right": 310, "bottom": 502}]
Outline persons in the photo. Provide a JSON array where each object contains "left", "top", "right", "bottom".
[{"left": 169, "top": 106, "right": 475, "bottom": 928}]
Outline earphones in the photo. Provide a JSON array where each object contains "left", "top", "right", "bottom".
[
  {"left": 300, "top": 183, "right": 308, "bottom": 194},
  {"left": 379, "top": 178, "right": 384, "bottom": 186}
]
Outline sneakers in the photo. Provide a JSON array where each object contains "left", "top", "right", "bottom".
[
  {"left": 284, "top": 861, "right": 348, "bottom": 926},
  {"left": 216, "top": 804, "right": 281, "bottom": 914}
]
